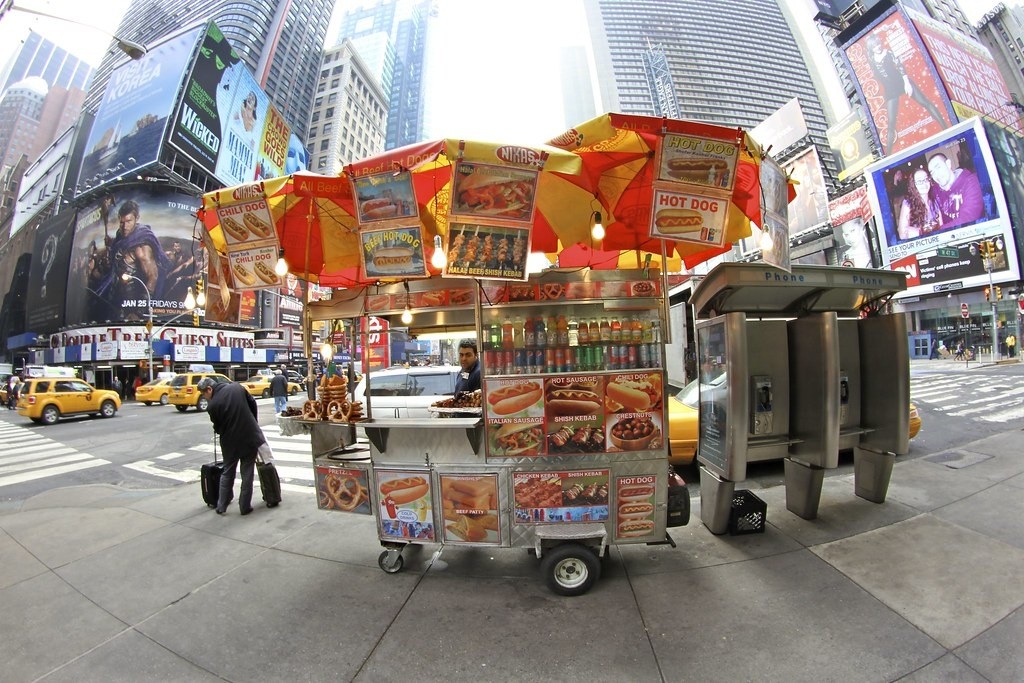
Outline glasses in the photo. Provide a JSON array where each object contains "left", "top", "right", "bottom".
[{"left": 914, "top": 178, "right": 929, "bottom": 185}]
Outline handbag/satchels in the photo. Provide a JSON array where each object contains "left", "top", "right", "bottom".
[{"left": 258, "top": 433, "right": 275, "bottom": 465}]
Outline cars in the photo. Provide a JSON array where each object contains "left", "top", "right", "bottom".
[
  {"left": 669, "top": 367, "right": 923, "bottom": 476},
  {"left": 0, "top": 381, "right": 24, "bottom": 405},
  {"left": 134, "top": 371, "right": 177, "bottom": 406},
  {"left": 341, "top": 370, "right": 363, "bottom": 384},
  {"left": 248, "top": 369, "right": 301, "bottom": 399}
]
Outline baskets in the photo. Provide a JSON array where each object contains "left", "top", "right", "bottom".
[{"left": 729, "top": 489, "right": 767, "bottom": 536}]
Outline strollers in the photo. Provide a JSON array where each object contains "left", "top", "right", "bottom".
[{"left": 963, "top": 349, "right": 976, "bottom": 361}]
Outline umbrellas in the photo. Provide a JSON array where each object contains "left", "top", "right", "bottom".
[{"left": 205, "top": 111, "right": 801, "bottom": 356}]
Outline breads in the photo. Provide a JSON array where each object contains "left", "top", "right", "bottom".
[
  {"left": 543, "top": 283, "right": 563, "bottom": 300},
  {"left": 319, "top": 472, "right": 369, "bottom": 512},
  {"left": 303, "top": 375, "right": 365, "bottom": 423},
  {"left": 442, "top": 477, "right": 498, "bottom": 543}
]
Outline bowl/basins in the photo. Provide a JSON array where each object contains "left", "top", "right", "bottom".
[
  {"left": 632, "top": 283, "right": 653, "bottom": 296},
  {"left": 609, "top": 418, "right": 657, "bottom": 451}
]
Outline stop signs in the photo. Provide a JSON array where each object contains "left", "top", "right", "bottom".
[{"left": 960, "top": 302, "right": 969, "bottom": 318}]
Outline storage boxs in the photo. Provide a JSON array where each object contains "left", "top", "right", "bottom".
[{"left": 727, "top": 490, "right": 767, "bottom": 536}]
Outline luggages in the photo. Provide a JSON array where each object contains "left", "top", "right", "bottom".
[
  {"left": 255, "top": 450, "right": 281, "bottom": 508},
  {"left": 201, "top": 431, "right": 234, "bottom": 509}
]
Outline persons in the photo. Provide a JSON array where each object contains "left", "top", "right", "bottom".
[
  {"left": 839, "top": 218, "right": 877, "bottom": 268},
  {"left": 930, "top": 339, "right": 938, "bottom": 359},
  {"left": 867, "top": 37, "right": 948, "bottom": 157},
  {"left": 281, "top": 273, "right": 302, "bottom": 297},
  {"left": 303, "top": 364, "right": 316, "bottom": 400},
  {"left": 197, "top": 377, "right": 266, "bottom": 515},
  {"left": 113, "top": 376, "right": 142, "bottom": 400},
  {"left": 323, "top": 363, "right": 357, "bottom": 381},
  {"left": 454, "top": 338, "right": 480, "bottom": 398},
  {"left": 270, "top": 369, "right": 288, "bottom": 413},
  {"left": 68, "top": 200, "right": 208, "bottom": 319},
  {"left": 898, "top": 153, "right": 983, "bottom": 239},
  {"left": 285, "top": 134, "right": 306, "bottom": 174},
  {"left": 1004, "top": 130, "right": 1024, "bottom": 233},
  {"left": 1006, "top": 333, "right": 1016, "bottom": 357},
  {"left": 281, "top": 364, "right": 288, "bottom": 401},
  {"left": 233, "top": 90, "right": 258, "bottom": 148},
  {"left": 953, "top": 340, "right": 965, "bottom": 360},
  {"left": 7, "top": 382, "right": 19, "bottom": 409}
]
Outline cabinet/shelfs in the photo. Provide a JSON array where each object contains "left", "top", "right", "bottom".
[{"left": 293, "top": 277, "right": 668, "bottom": 559}]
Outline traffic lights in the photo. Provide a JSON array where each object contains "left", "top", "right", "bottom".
[
  {"left": 196, "top": 279, "right": 204, "bottom": 298},
  {"left": 996, "top": 287, "right": 1002, "bottom": 302},
  {"left": 978, "top": 244, "right": 986, "bottom": 258},
  {"left": 193, "top": 311, "right": 199, "bottom": 327},
  {"left": 139, "top": 359, "right": 147, "bottom": 369},
  {"left": 985, "top": 289, "right": 989, "bottom": 302},
  {"left": 987, "top": 242, "right": 996, "bottom": 258}
]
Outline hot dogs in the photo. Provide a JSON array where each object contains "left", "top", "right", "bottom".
[
  {"left": 493, "top": 422, "right": 543, "bottom": 455},
  {"left": 360, "top": 198, "right": 396, "bottom": 219},
  {"left": 488, "top": 381, "right": 542, "bottom": 415},
  {"left": 369, "top": 288, "right": 474, "bottom": 310},
  {"left": 666, "top": 158, "right": 732, "bottom": 180},
  {"left": 380, "top": 477, "right": 429, "bottom": 504},
  {"left": 607, "top": 380, "right": 658, "bottom": 410},
  {"left": 619, "top": 485, "right": 655, "bottom": 537},
  {"left": 457, "top": 169, "right": 535, "bottom": 215},
  {"left": 547, "top": 389, "right": 603, "bottom": 412},
  {"left": 655, "top": 209, "right": 703, "bottom": 233},
  {"left": 373, "top": 248, "right": 415, "bottom": 271},
  {"left": 223, "top": 211, "right": 278, "bottom": 286}
]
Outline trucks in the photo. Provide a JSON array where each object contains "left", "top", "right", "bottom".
[{"left": 0, "top": 364, "right": 15, "bottom": 388}]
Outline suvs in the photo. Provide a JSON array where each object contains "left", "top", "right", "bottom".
[
  {"left": 17, "top": 367, "right": 122, "bottom": 424},
  {"left": 168, "top": 363, "right": 250, "bottom": 412},
  {"left": 286, "top": 370, "right": 307, "bottom": 391},
  {"left": 349, "top": 364, "right": 464, "bottom": 419}
]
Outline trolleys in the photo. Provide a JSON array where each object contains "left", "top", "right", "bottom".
[
  {"left": 936, "top": 348, "right": 947, "bottom": 359},
  {"left": 291, "top": 230, "right": 678, "bottom": 596}
]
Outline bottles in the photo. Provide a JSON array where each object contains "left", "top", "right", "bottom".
[
  {"left": 708, "top": 164, "right": 716, "bottom": 184},
  {"left": 514, "top": 314, "right": 523, "bottom": 348},
  {"left": 621, "top": 316, "right": 631, "bottom": 345},
  {"left": 535, "top": 317, "right": 547, "bottom": 348},
  {"left": 516, "top": 508, "right": 545, "bottom": 522},
  {"left": 502, "top": 315, "right": 513, "bottom": 350},
  {"left": 558, "top": 316, "right": 568, "bottom": 347},
  {"left": 600, "top": 317, "right": 610, "bottom": 345},
  {"left": 524, "top": 317, "right": 535, "bottom": 348},
  {"left": 632, "top": 315, "right": 642, "bottom": 345},
  {"left": 568, "top": 315, "right": 579, "bottom": 347},
  {"left": 588, "top": 317, "right": 600, "bottom": 345},
  {"left": 578, "top": 317, "right": 588, "bottom": 346},
  {"left": 490, "top": 314, "right": 502, "bottom": 350},
  {"left": 611, "top": 317, "right": 621, "bottom": 345},
  {"left": 383, "top": 520, "right": 433, "bottom": 540},
  {"left": 547, "top": 317, "right": 557, "bottom": 347},
  {"left": 642, "top": 315, "right": 652, "bottom": 344}
]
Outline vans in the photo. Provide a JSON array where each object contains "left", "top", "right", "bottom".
[{"left": 341, "top": 361, "right": 362, "bottom": 375}]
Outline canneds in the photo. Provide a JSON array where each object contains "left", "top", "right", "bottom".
[
  {"left": 715, "top": 171, "right": 730, "bottom": 187},
  {"left": 484, "top": 344, "right": 660, "bottom": 376},
  {"left": 395, "top": 200, "right": 411, "bottom": 215},
  {"left": 700, "top": 227, "right": 715, "bottom": 241}
]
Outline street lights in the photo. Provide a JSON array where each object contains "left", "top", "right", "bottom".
[
  {"left": 0, "top": 0, "right": 147, "bottom": 60},
  {"left": 122, "top": 274, "right": 152, "bottom": 382},
  {"left": 968, "top": 235, "right": 1003, "bottom": 362}
]
